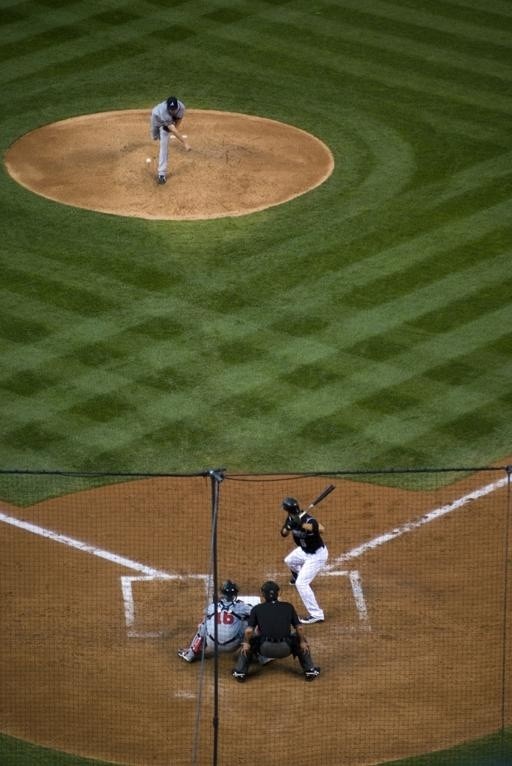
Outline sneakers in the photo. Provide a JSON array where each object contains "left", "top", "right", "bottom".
[
  {"left": 159, "top": 175, "right": 166, "bottom": 184},
  {"left": 305, "top": 667, "right": 320, "bottom": 682},
  {"left": 258, "top": 655, "right": 275, "bottom": 666},
  {"left": 178, "top": 648, "right": 195, "bottom": 663},
  {"left": 299, "top": 616, "right": 325, "bottom": 624},
  {"left": 289, "top": 579, "right": 296, "bottom": 585},
  {"left": 231, "top": 669, "right": 246, "bottom": 682}
]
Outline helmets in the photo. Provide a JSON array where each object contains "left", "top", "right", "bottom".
[
  {"left": 261, "top": 581, "right": 279, "bottom": 601},
  {"left": 221, "top": 579, "right": 239, "bottom": 595},
  {"left": 283, "top": 497, "right": 300, "bottom": 515}
]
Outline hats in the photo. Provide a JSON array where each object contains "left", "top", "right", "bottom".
[{"left": 167, "top": 97, "right": 178, "bottom": 110}]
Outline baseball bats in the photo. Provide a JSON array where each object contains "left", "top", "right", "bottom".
[{"left": 287, "top": 483, "right": 336, "bottom": 530}]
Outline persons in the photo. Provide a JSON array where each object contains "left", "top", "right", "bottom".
[
  {"left": 177, "top": 578, "right": 253, "bottom": 666},
  {"left": 279, "top": 495, "right": 328, "bottom": 626},
  {"left": 231, "top": 579, "right": 321, "bottom": 683},
  {"left": 150, "top": 95, "right": 193, "bottom": 184}
]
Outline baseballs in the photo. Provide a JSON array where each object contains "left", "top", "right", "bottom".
[{"left": 146, "top": 158, "right": 152, "bottom": 163}]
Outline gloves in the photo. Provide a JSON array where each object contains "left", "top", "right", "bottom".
[{"left": 286, "top": 520, "right": 306, "bottom": 533}]
